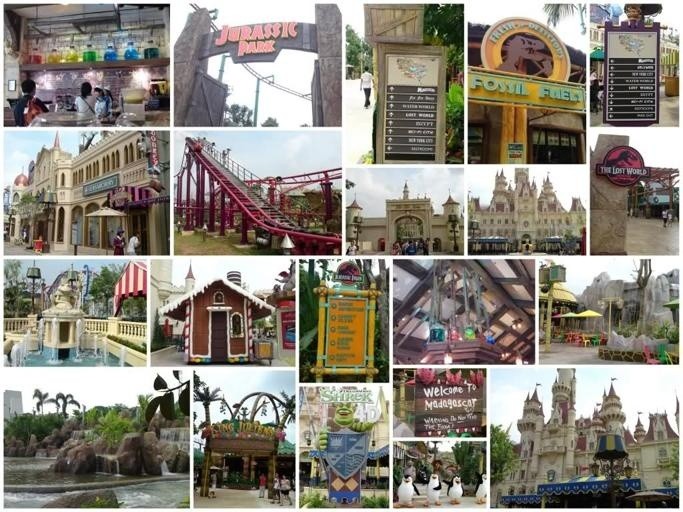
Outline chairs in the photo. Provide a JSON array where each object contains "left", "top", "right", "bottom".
[{"left": 553, "top": 328, "right": 679, "bottom": 365}]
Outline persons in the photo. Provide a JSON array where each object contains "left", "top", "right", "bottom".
[
  {"left": 661, "top": 209, "right": 667, "bottom": 226},
  {"left": 345, "top": 240, "right": 359, "bottom": 255},
  {"left": 126, "top": 230, "right": 143, "bottom": 254},
  {"left": 430, "top": 458, "right": 455, "bottom": 481},
  {"left": 206, "top": 469, "right": 218, "bottom": 498},
  {"left": 13, "top": 79, "right": 48, "bottom": 126},
  {"left": 75, "top": 82, "right": 96, "bottom": 115},
  {"left": 279, "top": 473, "right": 294, "bottom": 505},
  {"left": 391, "top": 236, "right": 432, "bottom": 255},
  {"left": 33, "top": 232, "right": 42, "bottom": 251},
  {"left": 104, "top": 85, "right": 113, "bottom": 116},
  {"left": 270, "top": 472, "right": 280, "bottom": 504},
  {"left": 522, "top": 244, "right": 532, "bottom": 255},
  {"left": 667, "top": 205, "right": 675, "bottom": 227},
  {"left": 112, "top": 227, "right": 126, "bottom": 254},
  {"left": 21, "top": 224, "right": 27, "bottom": 241},
  {"left": 259, "top": 472, "right": 267, "bottom": 499},
  {"left": 359, "top": 66, "right": 375, "bottom": 108},
  {"left": 193, "top": 469, "right": 198, "bottom": 491},
  {"left": 591, "top": 68, "right": 597, "bottom": 80},
  {"left": 94, "top": 83, "right": 106, "bottom": 116}
]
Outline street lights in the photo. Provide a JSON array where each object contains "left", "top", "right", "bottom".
[
  {"left": 468, "top": 218, "right": 480, "bottom": 254},
  {"left": 589, "top": 424, "right": 635, "bottom": 508},
  {"left": 27, "top": 259, "right": 42, "bottom": 314},
  {"left": 353, "top": 215, "right": 363, "bottom": 251},
  {"left": 63, "top": 263, "right": 80, "bottom": 289},
  {"left": 35, "top": 185, "right": 58, "bottom": 253},
  {"left": 447, "top": 213, "right": 461, "bottom": 251}
]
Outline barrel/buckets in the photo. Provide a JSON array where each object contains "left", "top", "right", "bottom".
[{"left": 665, "top": 76, "right": 679, "bottom": 96}]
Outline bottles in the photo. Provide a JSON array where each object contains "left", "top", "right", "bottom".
[{"left": 26, "top": 39, "right": 161, "bottom": 64}]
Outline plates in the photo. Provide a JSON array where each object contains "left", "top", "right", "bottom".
[{"left": 26, "top": 112, "right": 103, "bottom": 126}]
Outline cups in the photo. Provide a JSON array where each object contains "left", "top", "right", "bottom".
[
  {"left": 47, "top": 104, "right": 55, "bottom": 112},
  {"left": 113, "top": 112, "right": 137, "bottom": 127}
]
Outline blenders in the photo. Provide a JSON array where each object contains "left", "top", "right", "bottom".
[{"left": 118, "top": 87, "right": 149, "bottom": 125}]
